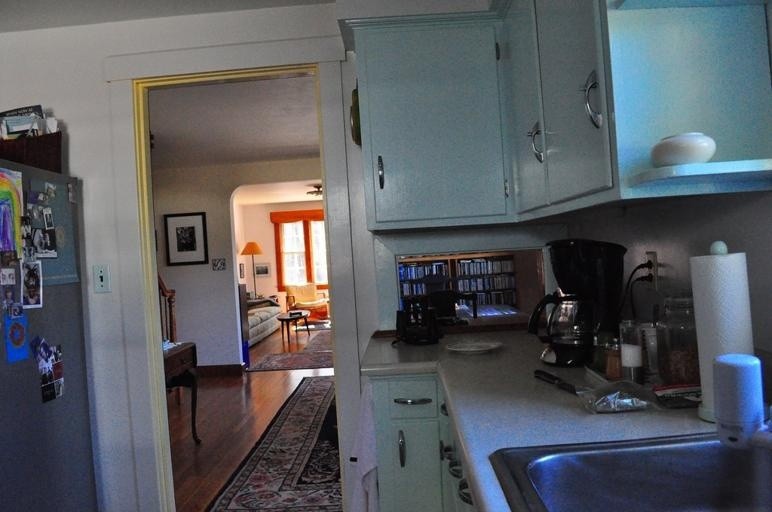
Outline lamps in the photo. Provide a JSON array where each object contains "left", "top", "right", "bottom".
[{"left": 241, "top": 242, "right": 265, "bottom": 298}]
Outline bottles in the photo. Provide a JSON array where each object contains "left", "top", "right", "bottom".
[
  {"left": 592, "top": 317, "right": 664, "bottom": 385},
  {"left": 657, "top": 297, "right": 696, "bottom": 388}
]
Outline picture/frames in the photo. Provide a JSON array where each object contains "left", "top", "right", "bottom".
[
  {"left": 163, "top": 210, "right": 209, "bottom": 266},
  {"left": 255, "top": 263, "right": 271, "bottom": 277}
]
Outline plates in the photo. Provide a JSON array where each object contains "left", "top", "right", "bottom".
[{"left": 446, "top": 339, "right": 503, "bottom": 355}]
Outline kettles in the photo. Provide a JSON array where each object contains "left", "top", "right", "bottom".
[{"left": 526, "top": 289, "right": 588, "bottom": 368}]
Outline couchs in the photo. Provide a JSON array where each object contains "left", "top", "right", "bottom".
[{"left": 248, "top": 298, "right": 280, "bottom": 346}]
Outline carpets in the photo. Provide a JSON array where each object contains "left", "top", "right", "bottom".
[
  {"left": 249, "top": 330, "right": 334, "bottom": 372},
  {"left": 207, "top": 376, "right": 341, "bottom": 510}
]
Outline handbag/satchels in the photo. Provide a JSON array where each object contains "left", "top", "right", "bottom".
[{"left": 0, "top": 116, "right": 62, "bottom": 174}]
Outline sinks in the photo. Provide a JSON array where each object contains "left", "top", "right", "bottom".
[{"left": 525, "top": 439, "right": 772, "bottom": 511}]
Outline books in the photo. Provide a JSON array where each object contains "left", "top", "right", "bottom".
[
  {"left": 398, "top": 256, "right": 518, "bottom": 311},
  {"left": 0, "top": 103, "right": 61, "bottom": 142}
]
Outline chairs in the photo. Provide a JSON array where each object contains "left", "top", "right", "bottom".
[{"left": 287, "top": 283, "right": 328, "bottom": 321}]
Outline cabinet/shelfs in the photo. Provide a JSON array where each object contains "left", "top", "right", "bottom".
[
  {"left": 500, "top": 2, "right": 768, "bottom": 221},
  {"left": 438, "top": 382, "right": 476, "bottom": 512},
  {"left": 356, "top": 28, "right": 509, "bottom": 230},
  {"left": 385, "top": 378, "right": 441, "bottom": 510}
]
